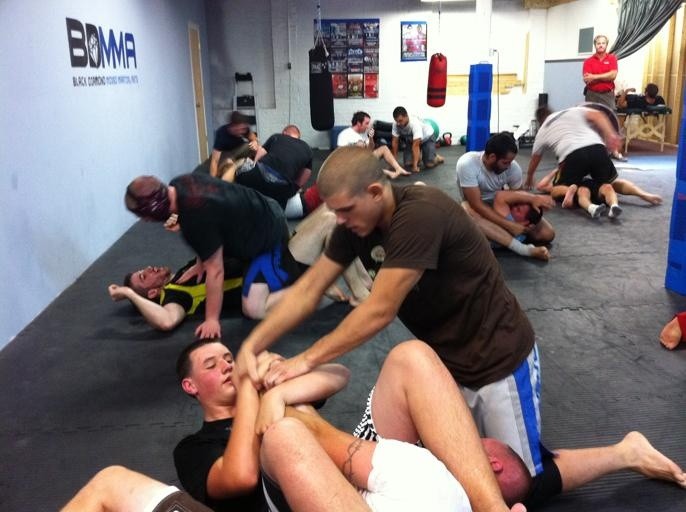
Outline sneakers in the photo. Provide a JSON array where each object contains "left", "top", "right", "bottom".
[{"left": 612, "top": 152, "right": 628, "bottom": 162}]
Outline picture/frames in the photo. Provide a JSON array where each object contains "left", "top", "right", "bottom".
[{"left": 399, "top": 21, "right": 428, "bottom": 62}]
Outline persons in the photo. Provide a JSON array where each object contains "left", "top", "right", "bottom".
[
  {"left": 62, "top": 147, "right": 686, "bottom": 511},
  {"left": 104, "top": 35, "right": 664, "bottom": 339}
]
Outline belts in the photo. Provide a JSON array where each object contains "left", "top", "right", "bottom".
[{"left": 588, "top": 87, "right": 615, "bottom": 95}]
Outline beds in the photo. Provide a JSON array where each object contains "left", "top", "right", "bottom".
[{"left": 612, "top": 103, "right": 673, "bottom": 157}]
